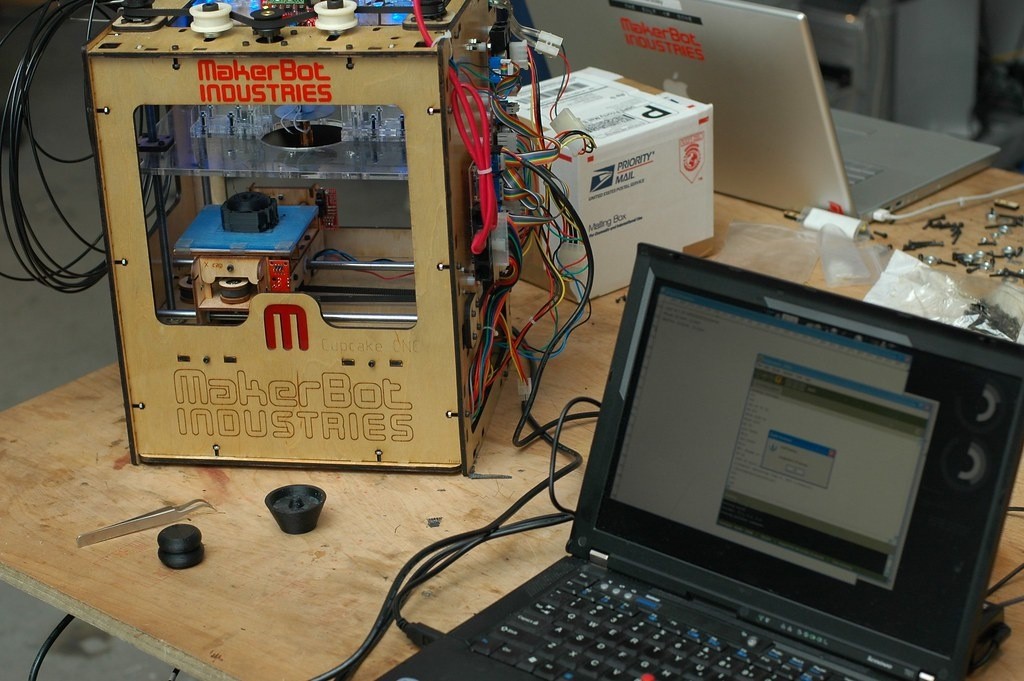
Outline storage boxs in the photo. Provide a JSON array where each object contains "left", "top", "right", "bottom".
[{"left": 508, "top": 65, "right": 714, "bottom": 304}]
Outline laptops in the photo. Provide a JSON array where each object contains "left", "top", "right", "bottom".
[
  {"left": 525, "top": 0, "right": 1000, "bottom": 221},
  {"left": 378, "top": 242, "right": 1024, "bottom": 681}
]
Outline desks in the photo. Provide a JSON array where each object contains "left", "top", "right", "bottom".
[{"left": 0, "top": 170, "right": 1024, "bottom": 681}]
